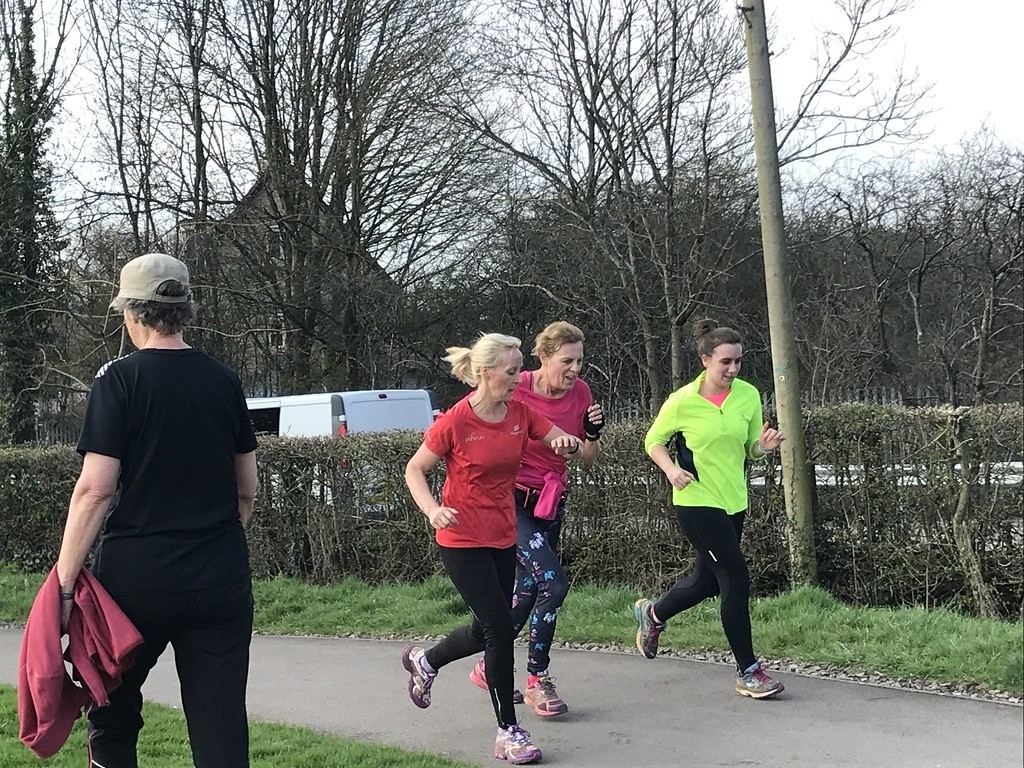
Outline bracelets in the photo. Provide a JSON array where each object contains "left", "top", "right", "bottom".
[
  {"left": 568, "top": 438, "right": 579, "bottom": 454},
  {"left": 757, "top": 445, "right": 766, "bottom": 454},
  {"left": 60, "top": 592, "right": 73, "bottom": 600},
  {"left": 584, "top": 432, "right": 600, "bottom": 442}
]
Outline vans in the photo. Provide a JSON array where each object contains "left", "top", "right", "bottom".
[{"left": 242, "top": 389, "right": 440, "bottom": 523}]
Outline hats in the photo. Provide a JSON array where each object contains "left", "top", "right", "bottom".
[{"left": 108, "top": 253, "right": 190, "bottom": 308}]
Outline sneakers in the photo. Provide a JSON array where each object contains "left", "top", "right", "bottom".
[
  {"left": 494, "top": 717, "right": 542, "bottom": 765},
  {"left": 402, "top": 644, "right": 438, "bottom": 708},
  {"left": 635, "top": 599, "right": 667, "bottom": 660},
  {"left": 735, "top": 661, "right": 785, "bottom": 698},
  {"left": 524, "top": 676, "right": 568, "bottom": 716},
  {"left": 469, "top": 656, "right": 524, "bottom": 704}
]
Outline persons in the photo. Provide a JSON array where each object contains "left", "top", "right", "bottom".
[
  {"left": 633, "top": 319, "right": 787, "bottom": 698},
  {"left": 406, "top": 333, "right": 585, "bottom": 764},
  {"left": 56, "top": 255, "right": 259, "bottom": 768},
  {"left": 470, "top": 320, "right": 606, "bottom": 716}
]
